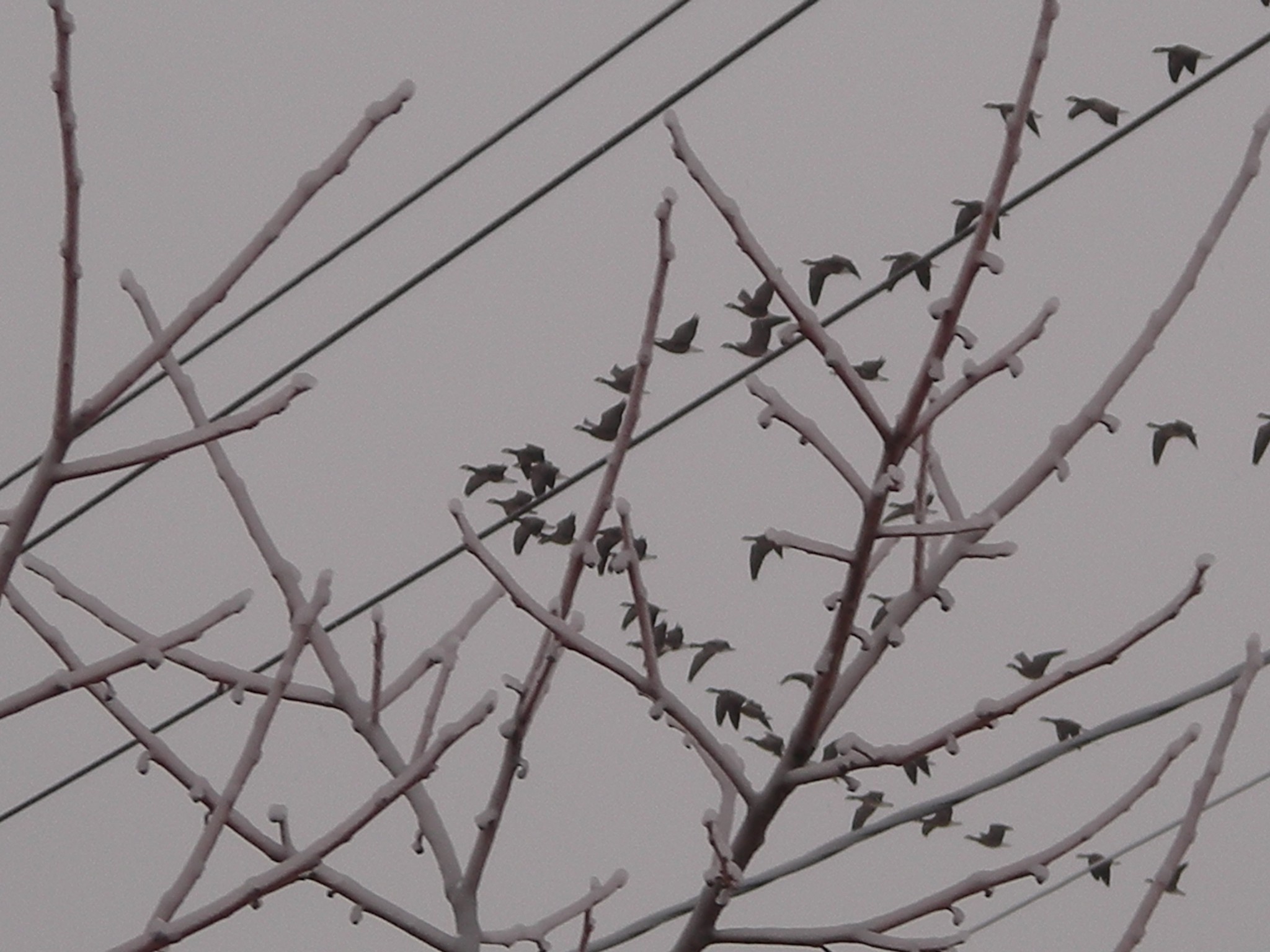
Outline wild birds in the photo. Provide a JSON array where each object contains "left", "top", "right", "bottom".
[{"left": 452, "top": 44, "right": 1270, "bottom": 893}]
